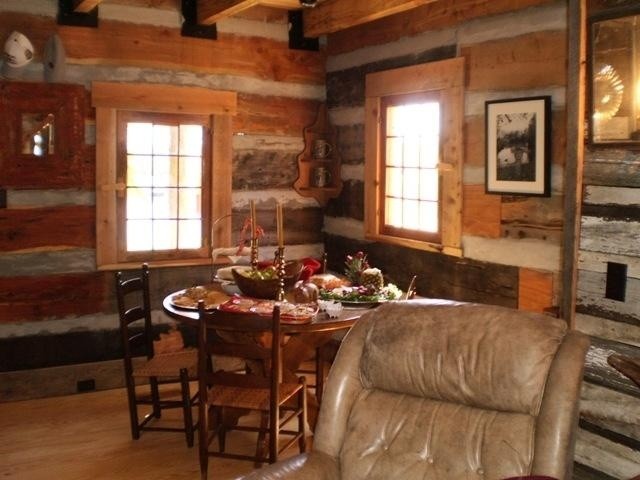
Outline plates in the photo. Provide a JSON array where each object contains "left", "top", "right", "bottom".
[
  {"left": 168, "top": 289, "right": 232, "bottom": 311},
  {"left": 326, "top": 290, "right": 404, "bottom": 304}
]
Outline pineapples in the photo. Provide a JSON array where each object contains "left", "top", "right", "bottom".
[{"left": 345, "top": 254, "right": 384, "bottom": 292}]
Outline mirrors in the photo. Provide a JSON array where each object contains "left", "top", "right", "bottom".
[{"left": 588, "top": 11, "right": 640, "bottom": 149}]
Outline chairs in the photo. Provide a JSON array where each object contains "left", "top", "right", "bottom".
[
  {"left": 197, "top": 299, "right": 306, "bottom": 480},
  {"left": 238, "top": 300, "right": 590, "bottom": 480},
  {"left": 297, "top": 254, "right": 329, "bottom": 404},
  {"left": 115, "top": 263, "right": 210, "bottom": 448}
]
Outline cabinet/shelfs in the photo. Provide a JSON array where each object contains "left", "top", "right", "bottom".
[{"left": 295, "top": 103, "right": 343, "bottom": 204}]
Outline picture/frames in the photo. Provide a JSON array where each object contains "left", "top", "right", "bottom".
[{"left": 482, "top": 95, "right": 555, "bottom": 200}]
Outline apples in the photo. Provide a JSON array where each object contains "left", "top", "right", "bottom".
[{"left": 241, "top": 265, "right": 279, "bottom": 280}]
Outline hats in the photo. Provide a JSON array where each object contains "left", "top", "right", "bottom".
[
  {"left": 1, "top": 30, "right": 33, "bottom": 79},
  {"left": 43, "top": 35, "right": 65, "bottom": 84}
]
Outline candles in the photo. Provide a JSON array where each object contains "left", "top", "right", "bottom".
[
  {"left": 250, "top": 201, "right": 258, "bottom": 240},
  {"left": 277, "top": 205, "right": 286, "bottom": 247}
]
